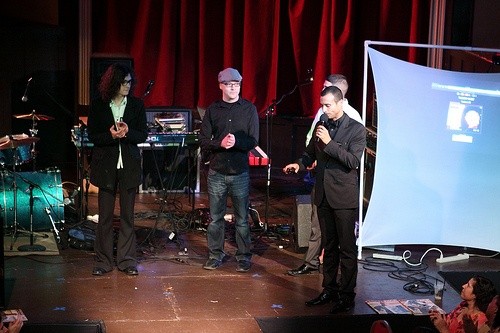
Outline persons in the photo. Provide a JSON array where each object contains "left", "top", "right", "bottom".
[
  {"left": 199, "top": 67, "right": 260, "bottom": 272},
  {"left": 429, "top": 275, "right": 496, "bottom": 333},
  {"left": 0, "top": 310, "right": 24, "bottom": 333},
  {"left": 287, "top": 74, "right": 363, "bottom": 275},
  {"left": 86, "top": 64, "right": 148, "bottom": 276},
  {"left": 283, "top": 87, "right": 367, "bottom": 313},
  {"left": 462, "top": 294, "right": 500, "bottom": 333}
]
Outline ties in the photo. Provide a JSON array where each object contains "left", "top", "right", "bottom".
[{"left": 321, "top": 122, "right": 336, "bottom": 153}]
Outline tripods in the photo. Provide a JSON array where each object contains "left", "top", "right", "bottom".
[
  {"left": 3, "top": 142, "right": 49, "bottom": 251},
  {"left": 249, "top": 77, "right": 309, "bottom": 248}
]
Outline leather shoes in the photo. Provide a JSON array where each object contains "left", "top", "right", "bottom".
[
  {"left": 118, "top": 265, "right": 139, "bottom": 276},
  {"left": 305, "top": 288, "right": 339, "bottom": 307},
  {"left": 203, "top": 258, "right": 224, "bottom": 271},
  {"left": 329, "top": 299, "right": 356, "bottom": 314},
  {"left": 236, "top": 259, "right": 252, "bottom": 274},
  {"left": 287, "top": 262, "right": 320, "bottom": 276},
  {"left": 92, "top": 267, "right": 113, "bottom": 276}
]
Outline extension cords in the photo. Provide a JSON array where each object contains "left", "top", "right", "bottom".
[
  {"left": 373, "top": 253, "right": 403, "bottom": 261},
  {"left": 436, "top": 254, "right": 469, "bottom": 263}
]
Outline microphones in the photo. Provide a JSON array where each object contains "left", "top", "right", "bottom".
[
  {"left": 315, "top": 114, "right": 329, "bottom": 142},
  {"left": 21, "top": 78, "right": 32, "bottom": 102},
  {"left": 144, "top": 79, "right": 154, "bottom": 96},
  {"left": 307, "top": 65, "right": 313, "bottom": 82}
]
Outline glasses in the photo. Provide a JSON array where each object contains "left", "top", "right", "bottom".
[
  {"left": 221, "top": 81, "right": 242, "bottom": 88},
  {"left": 120, "top": 79, "right": 136, "bottom": 87}
]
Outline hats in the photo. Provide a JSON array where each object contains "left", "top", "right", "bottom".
[{"left": 218, "top": 68, "right": 243, "bottom": 83}]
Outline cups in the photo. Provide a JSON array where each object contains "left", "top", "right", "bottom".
[{"left": 434, "top": 284, "right": 443, "bottom": 300}]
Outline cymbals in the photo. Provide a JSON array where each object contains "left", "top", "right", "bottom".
[
  {"left": 0, "top": 138, "right": 40, "bottom": 149},
  {"left": 12, "top": 113, "right": 55, "bottom": 121}
]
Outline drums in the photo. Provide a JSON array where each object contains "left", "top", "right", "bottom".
[
  {"left": 0, "top": 165, "right": 64, "bottom": 232},
  {"left": 0, "top": 133, "right": 30, "bottom": 164}
]
